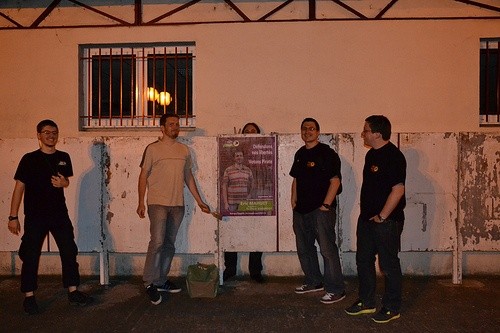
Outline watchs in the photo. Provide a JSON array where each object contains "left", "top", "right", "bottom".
[
  {"left": 9, "top": 215, "right": 18, "bottom": 220},
  {"left": 322, "top": 203, "right": 330, "bottom": 208}
]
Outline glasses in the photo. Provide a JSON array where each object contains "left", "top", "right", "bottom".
[
  {"left": 300, "top": 127, "right": 317, "bottom": 131},
  {"left": 362, "top": 128, "right": 372, "bottom": 133},
  {"left": 40, "top": 130, "right": 58, "bottom": 135}
]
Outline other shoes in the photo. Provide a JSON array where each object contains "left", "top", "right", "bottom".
[
  {"left": 223, "top": 269, "right": 236, "bottom": 282},
  {"left": 294, "top": 282, "right": 325, "bottom": 294},
  {"left": 250, "top": 271, "right": 266, "bottom": 283},
  {"left": 68, "top": 290, "right": 94, "bottom": 307},
  {"left": 23, "top": 295, "right": 38, "bottom": 315},
  {"left": 156, "top": 281, "right": 184, "bottom": 292},
  {"left": 371, "top": 305, "right": 401, "bottom": 323},
  {"left": 146, "top": 285, "right": 165, "bottom": 301},
  {"left": 344, "top": 299, "right": 376, "bottom": 315},
  {"left": 319, "top": 290, "right": 347, "bottom": 304}
]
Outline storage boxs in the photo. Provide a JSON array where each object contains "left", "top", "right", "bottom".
[{"left": 187, "top": 262, "right": 220, "bottom": 298}]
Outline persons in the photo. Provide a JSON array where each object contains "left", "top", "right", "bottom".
[
  {"left": 222, "top": 123, "right": 268, "bottom": 284},
  {"left": 344, "top": 115, "right": 407, "bottom": 323},
  {"left": 288, "top": 118, "right": 347, "bottom": 304},
  {"left": 221, "top": 150, "right": 254, "bottom": 212},
  {"left": 137, "top": 113, "right": 210, "bottom": 305},
  {"left": 8, "top": 119, "right": 94, "bottom": 316}
]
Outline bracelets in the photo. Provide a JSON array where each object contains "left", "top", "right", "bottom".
[{"left": 377, "top": 213, "right": 384, "bottom": 221}]
